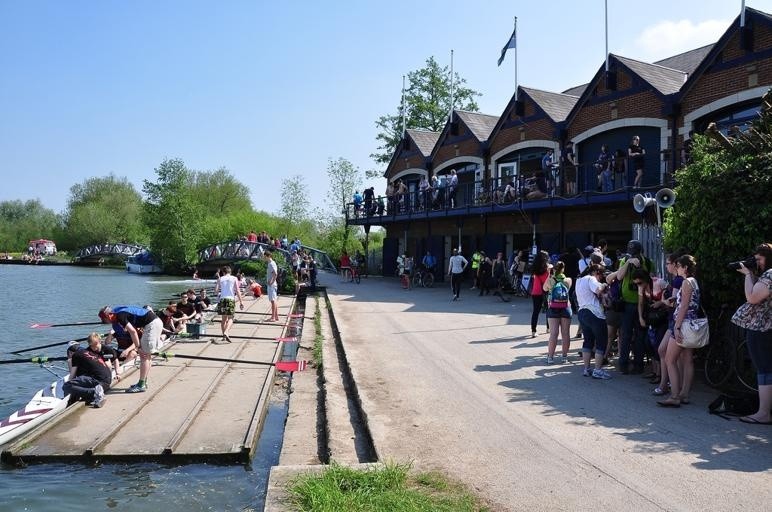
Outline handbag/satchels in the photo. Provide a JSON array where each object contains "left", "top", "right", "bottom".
[
  {"left": 674, "top": 316, "right": 710, "bottom": 349},
  {"left": 642, "top": 303, "right": 668, "bottom": 324}
]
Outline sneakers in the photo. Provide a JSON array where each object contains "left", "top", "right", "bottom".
[
  {"left": 592, "top": 368, "right": 611, "bottom": 379},
  {"left": 125, "top": 383, "right": 148, "bottom": 393},
  {"left": 93, "top": 392, "right": 108, "bottom": 409},
  {"left": 583, "top": 367, "right": 592, "bottom": 377},
  {"left": 621, "top": 367, "right": 670, "bottom": 394},
  {"left": 561, "top": 355, "right": 569, "bottom": 364},
  {"left": 547, "top": 353, "right": 555, "bottom": 364}
]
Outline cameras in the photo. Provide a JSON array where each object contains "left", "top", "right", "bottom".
[{"left": 726, "top": 255, "right": 757, "bottom": 272}]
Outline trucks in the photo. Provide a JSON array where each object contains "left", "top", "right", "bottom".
[{"left": 29, "top": 239, "right": 59, "bottom": 256}]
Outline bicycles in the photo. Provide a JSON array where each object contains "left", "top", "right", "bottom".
[
  {"left": 496, "top": 262, "right": 528, "bottom": 303},
  {"left": 412, "top": 264, "right": 437, "bottom": 289},
  {"left": 732, "top": 337, "right": 761, "bottom": 393},
  {"left": 699, "top": 301, "right": 736, "bottom": 387},
  {"left": 344, "top": 265, "right": 362, "bottom": 284}
]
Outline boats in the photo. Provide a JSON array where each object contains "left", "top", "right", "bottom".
[
  {"left": 123, "top": 251, "right": 166, "bottom": 275},
  {"left": 0, "top": 300, "right": 223, "bottom": 445}
]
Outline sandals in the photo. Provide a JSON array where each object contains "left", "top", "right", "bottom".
[{"left": 657, "top": 395, "right": 689, "bottom": 408}]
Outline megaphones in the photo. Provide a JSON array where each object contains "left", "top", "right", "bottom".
[
  {"left": 655, "top": 187, "right": 676, "bottom": 208},
  {"left": 633, "top": 193, "right": 655, "bottom": 213}
]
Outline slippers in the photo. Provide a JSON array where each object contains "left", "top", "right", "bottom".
[{"left": 739, "top": 415, "right": 771, "bottom": 426}]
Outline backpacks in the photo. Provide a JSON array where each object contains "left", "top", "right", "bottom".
[{"left": 548, "top": 276, "right": 570, "bottom": 309}]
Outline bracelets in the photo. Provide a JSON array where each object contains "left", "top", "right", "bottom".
[
  {"left": 745, "top": 273, "right": 752, "bottom": 276},
  {"left": 497, "top": 29, "right": 516, "bottom": 66}
]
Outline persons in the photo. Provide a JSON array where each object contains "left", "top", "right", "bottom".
[
  {"left": 2, "top": 248, "right": 44, "bottom": 264},
  {"left": 731, "top": 243, "right": 772, "bottom": 425}
]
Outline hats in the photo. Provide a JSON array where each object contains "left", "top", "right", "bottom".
[
  {"left": 98, "top": 305, "right": 112, "bottom": 320},
  {"left": 66, "top": 338, "right": 79, "bottom": 355}
]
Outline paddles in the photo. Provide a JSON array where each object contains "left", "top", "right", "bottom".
[
  {"left": 29, "top": 322, "right": 108, "bottom": 329},
  {"left": 204, "top": 305, "right": 303, "bottom": 318},
  {"left": 152, "top": 351, "right": 306, "bottom": 372},
  {"left": 180, "top": 334, "right": 297, "bottom": 342},
  {"left": 211, "top": 321, "right": 302, "bottom": 330}
]
